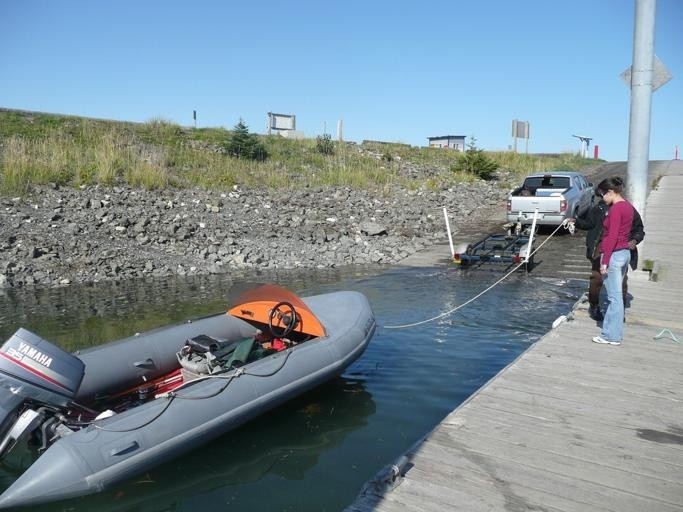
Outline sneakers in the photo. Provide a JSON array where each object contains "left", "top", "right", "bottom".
[
  {"left": 588, "top": 307, "right": 604, "bottom": 321},
  {"left": 592, "top": 336, "right": 621, "bottom": 345}
]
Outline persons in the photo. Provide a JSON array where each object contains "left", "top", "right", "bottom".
[
  {"left": 576, "top": 185, "right": 646, "bottom": 321},
  {"left": 593, "top": 176, "right": 634, "bottom": 346}
]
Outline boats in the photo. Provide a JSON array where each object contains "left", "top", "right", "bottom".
[{"left": 0, "top": 287, "right": 376, "bottom": 512}]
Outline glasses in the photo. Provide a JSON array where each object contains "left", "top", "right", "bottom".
[{"left": 600, "top": 191, "right": 608, "bottom": 198}]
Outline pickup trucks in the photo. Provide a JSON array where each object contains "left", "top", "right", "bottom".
[{"left": 506, "top": 171, "right": 596, "bottom": 235}]
